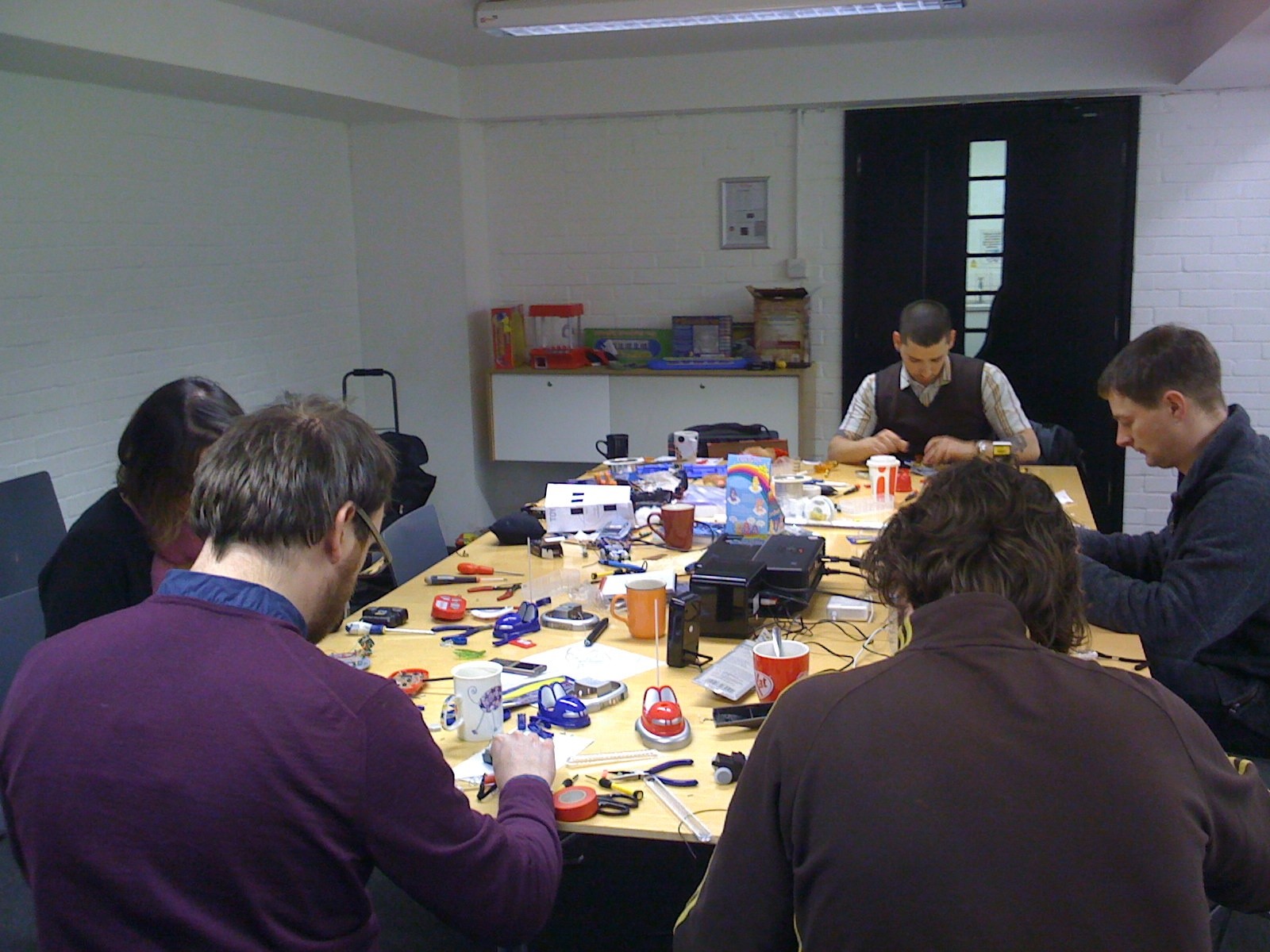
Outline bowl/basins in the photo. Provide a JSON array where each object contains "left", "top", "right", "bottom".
[
  {"left": 602, "top": 457, "right": 645, "bottom": 481},
  {"left": 773, "top": 475, "right": 811, "bottom": 498}
]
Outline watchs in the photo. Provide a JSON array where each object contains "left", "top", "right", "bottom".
[{"left": 972, "top": 439, "right": 986, "bottom": 459}]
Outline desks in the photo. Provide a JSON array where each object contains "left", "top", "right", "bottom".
[{"left": 325, "top": 463, "right": 1153, "bottom": 863}]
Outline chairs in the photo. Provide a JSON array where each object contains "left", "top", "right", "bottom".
[{"left": 381, "top": 502, "right": 448, "bottom": 587}]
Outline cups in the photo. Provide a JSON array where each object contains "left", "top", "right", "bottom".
[
  {"left": 674, "top": 430, "right": 699, "bottom": 463},
  {"left": 441, "top": 659, "right": 504, "bottom": 742},
  {"left": 866, "top": 455, "right": 901, "bottom": 509},
  {"left": 752, "top": 640, "right": 810, "bottom": 703},
  {"left": 647, "top": 502, "right": 695, "bottom": 552},
  {"left": 803, "top": 484, "right": 821, "bottom": 499},
  {"left": 595, "top": 434, "right": 629, "bottom": 468},
  {"left": 610, "top": 579, "right": 667, "bottom": 640}
]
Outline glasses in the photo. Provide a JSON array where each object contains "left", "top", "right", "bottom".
[{"left": 352, "top": 500, "right": 394, "bottom": 580}]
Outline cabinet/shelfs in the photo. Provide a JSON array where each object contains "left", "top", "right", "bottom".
[{"left": 485, "top": 362, "right": 818, "bottom": 463}]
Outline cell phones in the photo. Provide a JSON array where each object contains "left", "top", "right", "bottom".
[{"left": 491, "top": 658, "right": 546, "bottom": 677}]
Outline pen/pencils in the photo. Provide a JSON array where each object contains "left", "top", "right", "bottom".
[{"left": 591, "top": 559, "right": 646, "bottom": 579}]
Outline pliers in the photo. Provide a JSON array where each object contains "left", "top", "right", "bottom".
[
  {"left": 467, "top": 582, "right": 523, "bottom": 601},
  {"left": 430, "top": 624, "right": 495, "bottom": 641},
  {"left": 607, "top": 759, "right": 698, "bottom": 786}
]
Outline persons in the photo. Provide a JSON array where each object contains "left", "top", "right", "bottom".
[
  {"left": 674, "top": 454, "right": 1270, "bottom": 952},
  {"left": 1077, "top": 324, "right": 1270, "bottom": 786},
  {"left": 825, "top": 299, "right": 1041, "bottom": 466},
  {"left": 38, "top": 375, "right": 248, "bottom": 641},
  {"left": 0, "top": 392, "right": 562, "bottom": 952}
]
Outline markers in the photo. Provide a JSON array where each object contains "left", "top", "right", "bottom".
[{"left": 584, "top": 617, "right": 610, "bottom": 646}]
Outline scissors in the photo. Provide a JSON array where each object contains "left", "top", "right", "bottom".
[{"left": 596, "top": 793, "right": 638, "bottom": 816}]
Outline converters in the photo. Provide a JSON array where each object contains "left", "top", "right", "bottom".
[{"left": 825, "top": 597, "right": 868, "bottom": 621}]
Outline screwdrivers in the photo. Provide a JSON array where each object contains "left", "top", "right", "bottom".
[
  {"left": 837, "top": 483, "right": 860, "bottom": 499},
  {"left": 344, "top": 622, "right": 435, "bottom": 635},
  {"left": 585, "top": 774, "right": 644, "bottom": 800},
  {"left": 424, "top": 574, "right": 508, "bottom": 585},
  {"left": 553, "top": 774, "right": 579, "bottom": 793},
  {"left": 457, "top": 561, "right": 526, "bottom": 577}
]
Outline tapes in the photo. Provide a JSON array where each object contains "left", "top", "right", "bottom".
[{"left": 552, "top": 785, "right": 598, "bottom": 822}]
